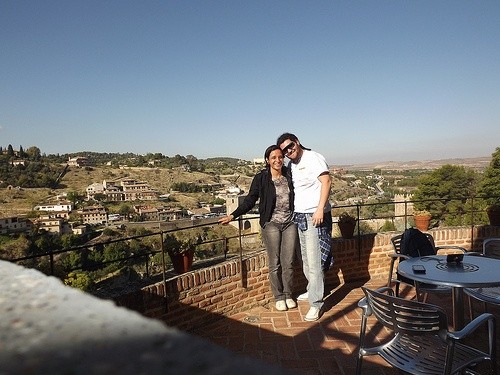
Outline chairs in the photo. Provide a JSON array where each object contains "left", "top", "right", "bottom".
[
  {"left": 355, "top": 286, "right": 500, "bottom": 375},
  {"left": 385, "top": 228, "right": 472, "bottom": 331},
  {"left": 460, "top": 237, "right": 500, "bottom": 322}
]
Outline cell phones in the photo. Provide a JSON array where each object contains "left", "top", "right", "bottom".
[{"left": 412, "top": 264, "right": 426, "bottom": 272}]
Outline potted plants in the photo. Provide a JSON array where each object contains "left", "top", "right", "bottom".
[
  {"left": 487, "top": 204, "right": 500, "bottom": 226},
  {"left": 413, "top": 209, "right": 432, "bottom": 231},
  {"left": 164, "top": 227, "right": 209, "bottom": 274},
  {"left": 338, "top": 212, "right": 356, "bottom": 239}
]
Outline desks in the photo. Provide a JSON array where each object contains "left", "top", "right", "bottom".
[{"left": 397, "top": 254, "right": 500, "bottom": 330}]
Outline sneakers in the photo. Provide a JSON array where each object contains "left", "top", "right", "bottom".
[
  {"left": 305, "top": 307, "right": 323, "bottom": 321},
  {"left": 295, "top": 291, "right": 309, "bottom": 300},
  {"left": 275, "top": 298, "right": 297, "bottom": 311}
]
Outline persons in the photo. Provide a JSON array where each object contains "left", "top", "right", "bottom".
[
  {"left": 218, "top": 145, "right": 298, "bottom": 311},
  {"left": 277, "top": 133, "right": 333, "bottom": 322}
]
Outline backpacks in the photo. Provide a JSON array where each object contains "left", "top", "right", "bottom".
[{"left": 399, "top": 227, "right": 438, "bottom": 288}]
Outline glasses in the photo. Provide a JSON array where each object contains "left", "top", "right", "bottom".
[{"left": 279, "top": 141, "right": 295, "bottom": 154}]
